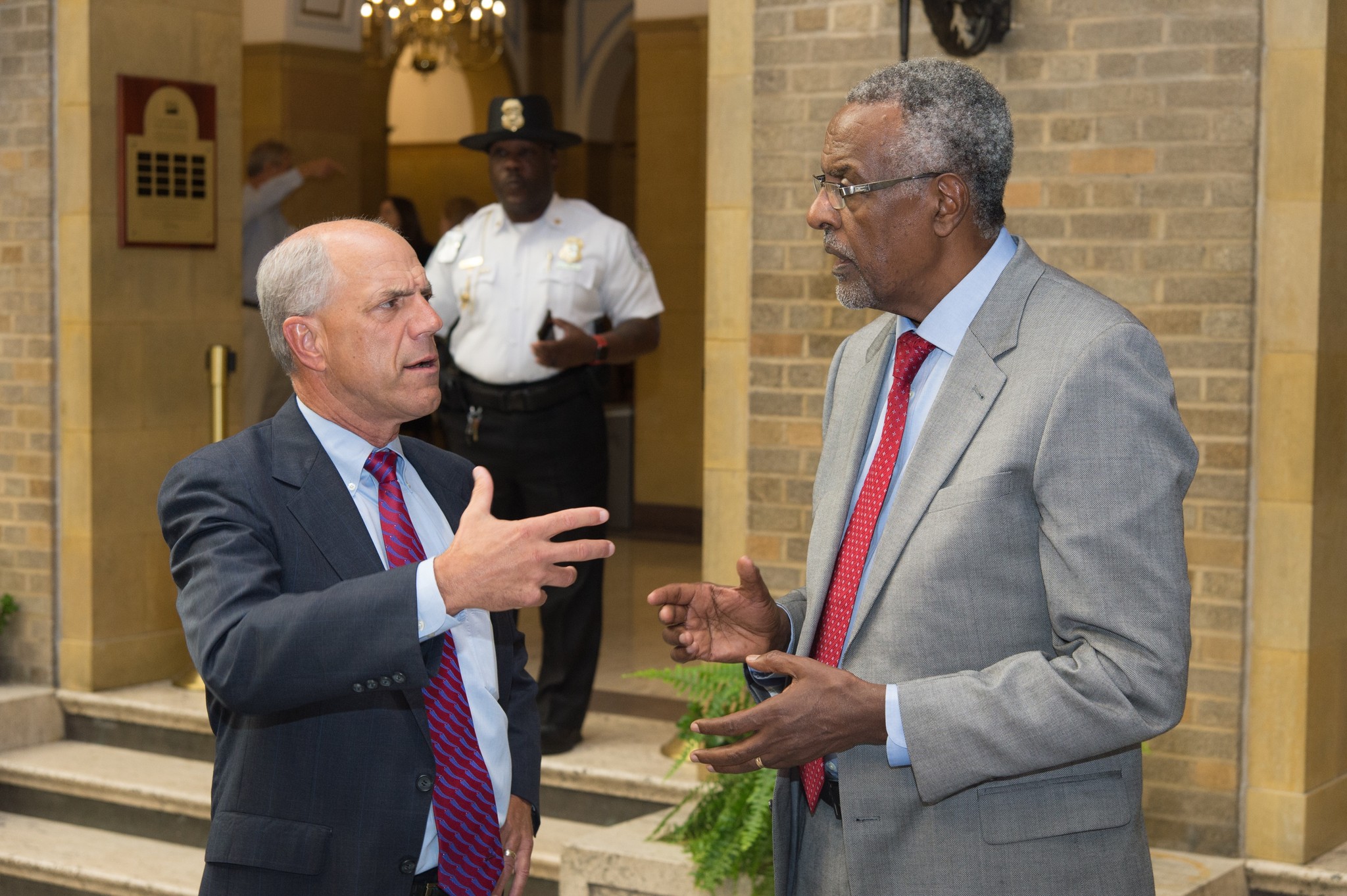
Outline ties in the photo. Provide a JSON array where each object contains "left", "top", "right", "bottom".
[
  {"left": 798, "top": 331, "right": 936, "bottom": 813},
  {"left": 363, "top": 450, "right": 504, "bottom": 896}
]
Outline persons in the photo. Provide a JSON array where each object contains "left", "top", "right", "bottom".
[
  {"left": 377, "top": 195, "right": 433, "bottom": 267},
  {"left": 422, "top": 93, "right": 668, "bottom": 759},
  {"left": 647, "top": 57, "right": 1202, "bottom": 896},
  {"left": 238, "top": 140, "right": 346, "bottom": 435},
  {"left": 155, "top": 216, "right": 616, "bottom": 896}
]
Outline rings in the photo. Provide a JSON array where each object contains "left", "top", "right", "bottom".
[
  {"left": 503, "top": 849, "right": 517, "bottom": 860},
  {"left": 755, "top": 756, "right": 768, "bottom": 768}
]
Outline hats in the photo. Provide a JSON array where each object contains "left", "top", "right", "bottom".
[{"left": 457, "top": 94, "right": 583, "bottom": 154}]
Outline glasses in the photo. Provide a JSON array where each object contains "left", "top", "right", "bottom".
[{"left": 812, "top": 172, "right": 944, "bottom": 209}]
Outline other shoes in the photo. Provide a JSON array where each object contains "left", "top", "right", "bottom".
[{"left": 539, "top": 728, "right": 582, "bottom": 755}]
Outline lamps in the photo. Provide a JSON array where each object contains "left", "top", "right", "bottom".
[{"left": 359, "top": 0, "right": 508, "bottom": 77}]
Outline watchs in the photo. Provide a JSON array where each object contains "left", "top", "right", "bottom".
[{"left": 589, "top": 334, "right": 609, "bottom": 366}]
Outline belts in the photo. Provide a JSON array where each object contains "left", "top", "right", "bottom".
[{"left": 440, "top": 367, "right": 605, "bottom": 411}]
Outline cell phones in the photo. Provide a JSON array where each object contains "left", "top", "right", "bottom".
[{"left": 536, "top": 310, "right": 557, "bottom": 341}]
